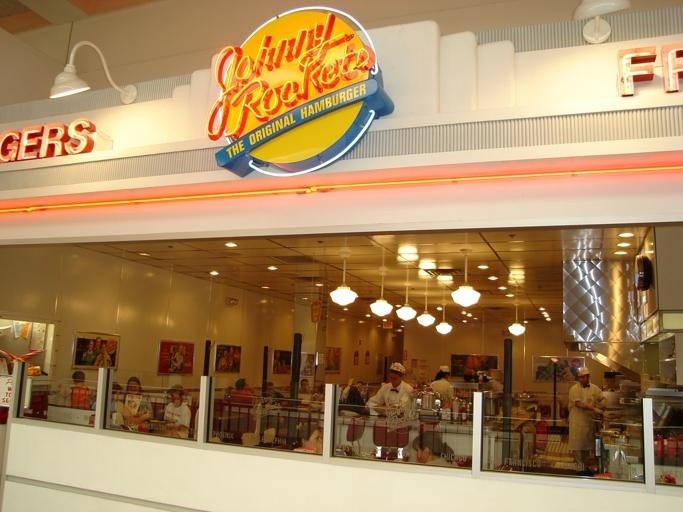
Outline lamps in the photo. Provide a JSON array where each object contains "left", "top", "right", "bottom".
[
  {"left": 508, "top": 303, "right": 526, "bottom": 337},
  {"left": 330, "top": 249, "right": 481, "bottom": 335},
  {"left": 50, "top": 40, "right": 138, "bottom": 105}
]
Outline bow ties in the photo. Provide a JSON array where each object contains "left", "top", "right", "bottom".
[
  {"left": 390, "top": 388, "right": 399, "bottom": 395},
  {"left": 582, "top": 384, "right": 591, "bottom": 388}
]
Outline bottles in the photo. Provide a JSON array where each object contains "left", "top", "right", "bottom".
[
  {"left": 439, "top": 394, "right": 470, "bottom": 422},
  {"left": 602, "top": 412, "right": 608, "bottom": 431},
  {"left": 421, "top": 386, "right": 433, "bottom": 409},
  {"left": 463, "top": 368, "right": 503, "bottom": 382},
  {"left": 519, "top": 391, "right": 531, "bottom": 398},
  {"left": 26, "top": 364, "right": 40, "bottom": 376}
]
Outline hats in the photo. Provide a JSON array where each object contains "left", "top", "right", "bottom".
[
  {"left": 577, "top": 367, "right": 591, "bottom": 377},
  {"left": 390, "top": 362, "right": 405, "bottom": 374},
  {"left": 439, "top": 365, "right": 451, "bottom": 373}
]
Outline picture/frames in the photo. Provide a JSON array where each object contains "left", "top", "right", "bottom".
[
  {"left": 326, "top": 346, "right": 342, "bottom": 373},
  {"left": 71, "top": 331, "right": 120, "bottom": 371},
  {"left": 300, "top": 352, "right": 316, "bottom": 377},
  {"left": 532, "top": 356, "right": 585, "bottom": 383},
  {"left": 450, "top": 353, "right": 500, "bottom": 379}
]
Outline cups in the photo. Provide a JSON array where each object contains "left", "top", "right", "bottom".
[
  {"left": 619, "top": 379, "right": 640, "bottom": 404},
  {"left": 482, "top": 398, "right": 496, "bottom": 416}
]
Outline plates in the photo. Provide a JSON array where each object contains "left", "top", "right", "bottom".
[{"left": 416, "top": 409, "right": 439, "bottom": 422}]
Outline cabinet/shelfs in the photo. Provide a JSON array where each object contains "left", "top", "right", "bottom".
[{"left": 635, "top": 223, "right": 683, "bottom": 325}]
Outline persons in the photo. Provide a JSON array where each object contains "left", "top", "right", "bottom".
[
  {"left": 82, "top": 337, "right": 112, "bottom": 367},
  {"left": 343, "top": 387, "right": 365, "bottom": 406},
  {"left": 231, "top": 378, "right": 251, "bottom": 395},
  {"left": 293, "top": 425, "right": 323, "bottom": 455},
  {"left": 168, "top": 344, "right": 187, "bottom": 372},
  {"left": 301, "top": 380, "right": 310, "bottom": 393},
  {"left": 430, "top": 365, "right": 453, "bottom": 407},
  {"left": 218, "top": 346, "right": 240, "bottom": 369},
  {"left": 71, "top": 371, "right": 91, "bottom": 410},
  {"left": 567, "top": 367, "right": 607, "bottom": 468},
  {"left": 412, "top": 431, "right": 461, "bottom": 468},
  {"left": 112, "top": 377, "right": 192, "bottom": 440},
  {"left": 365, "top": 362, "right": 414, "bottom": 416},
  {"left": 267, "top": 382, "right": 284, "bottom": 398}
]
